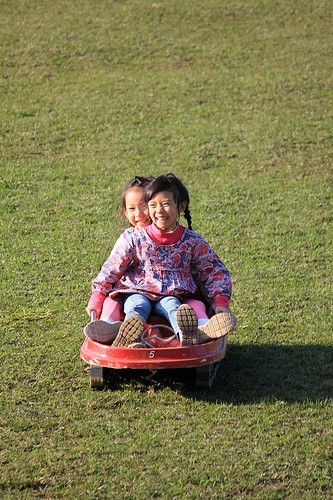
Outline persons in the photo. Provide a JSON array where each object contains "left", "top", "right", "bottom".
[
  {"left": 82, "top": 176, "right": 237, "bottom": 344},
  {"left": 85, "top": 173, "right": 232, "bottom": 348}
]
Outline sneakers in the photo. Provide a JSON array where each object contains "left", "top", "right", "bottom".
[
  {"left": 111, "top": 315, "right": 144, "bottom": 350},
  {"left": 83, "top": 320, "right": 124, "bottom": 343},
  {"left": 199, "top": 313, "right": 238, "bottom": 344},
  {"left": 176, "top": 303, "right": 200, "bottom": 347}
]
